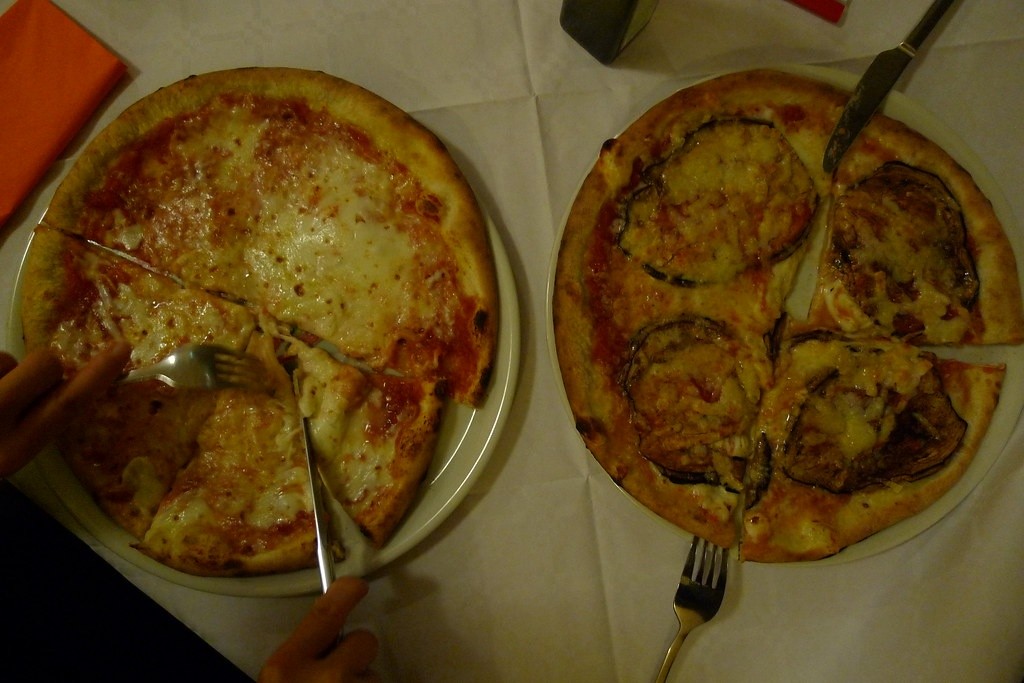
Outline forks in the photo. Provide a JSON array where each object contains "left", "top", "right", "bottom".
[
  {"left": 655, "top": 536, "right": 729, "bottom": 683},
  {"left": 21, "top": 343, "right": 268, "bottom": 394}
]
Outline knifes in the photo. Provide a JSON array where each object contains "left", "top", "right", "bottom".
[
  {"left": 301, "top": 417, "right": 336, "bottom": 597},
  {"left": 822, "top": 0, "right": 955, "bottom": 172}
]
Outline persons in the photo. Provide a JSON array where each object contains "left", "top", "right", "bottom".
[{"left": 0, "top": 336, "right": 382, "bottom": 682}]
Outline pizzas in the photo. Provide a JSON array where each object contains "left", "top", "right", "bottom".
[
  {"left": 19, "top": 66, "right": 499, "bottom": 581},
  {"left": 550, "top": 69, "right": 1024, "bottom": 566}
]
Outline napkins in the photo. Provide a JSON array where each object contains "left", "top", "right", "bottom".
[{"left": 0, "top": 0, "right": 129, "bottom": 233}]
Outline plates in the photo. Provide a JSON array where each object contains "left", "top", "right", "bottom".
[
  {"left": 8, "top": 184, "right": 524, "bottom": 604},
  {"left": 544, "top": 64, "right": 1024, "bottom": 572}
]
